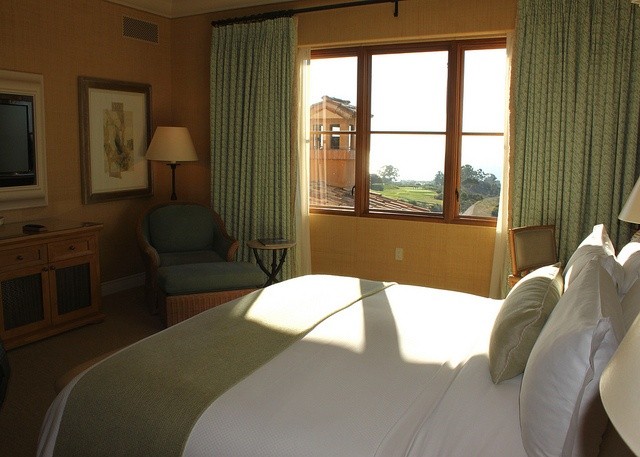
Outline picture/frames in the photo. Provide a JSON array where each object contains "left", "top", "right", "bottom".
[{"left": 77, "top": 76, "right": 154, "bottom": 206}]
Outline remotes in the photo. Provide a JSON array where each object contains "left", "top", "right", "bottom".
[{"left": 21, "top": 223, "right": 47, "bottom": 232}]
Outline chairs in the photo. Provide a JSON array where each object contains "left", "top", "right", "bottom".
[
  {"left": 508, "top": 225, "right": 565, "bottom": 290},
  {"left": 136, "top": 200, "right": 239, "bottom": 315}
]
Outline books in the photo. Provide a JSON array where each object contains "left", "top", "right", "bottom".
[{"left": 257, "top": 237, "right": 289, "bottom": 246}]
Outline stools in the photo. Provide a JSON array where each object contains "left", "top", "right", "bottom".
[{"left": 158, "top": 261, "right": 259, "bottom": 328}]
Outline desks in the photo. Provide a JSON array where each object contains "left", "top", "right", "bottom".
[{"left": 247, "top": 238, "right": 295, "bottom": 288}]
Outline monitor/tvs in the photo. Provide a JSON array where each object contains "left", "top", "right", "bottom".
[{"left": 0, "top": 80, "right": 45, "bottom": 198}]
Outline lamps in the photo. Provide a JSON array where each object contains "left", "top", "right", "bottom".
[{"left": 146, "top": 127, "right": 197, "bottom": 201}]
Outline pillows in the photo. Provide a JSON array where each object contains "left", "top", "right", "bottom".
[
  {"left": 519, "top": 258, "right": 625, "bottom": 457},
  {"left": 563, "top": 223, "right": 622, "bottom": 295},
  {"left": 489, "top": 262, "right": 563, "bottom": 384},
  {"left": 615, "top": 236, "right": 640, "bottom": 299}
]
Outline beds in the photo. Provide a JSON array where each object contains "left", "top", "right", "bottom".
[{"left": 37, "top": 275, "right": 640, "bottom": 457}]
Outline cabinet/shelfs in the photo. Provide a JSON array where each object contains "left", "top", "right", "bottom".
[{"left": 0, "top": 219, "right": 105, "bottom": 352}]
